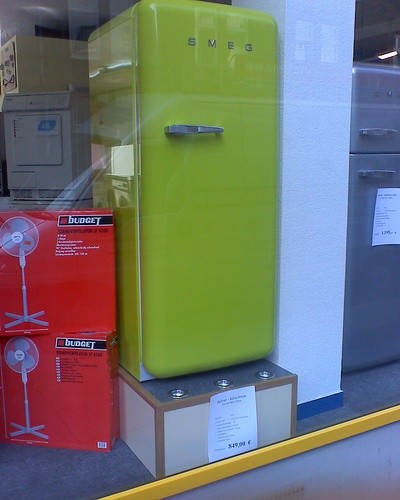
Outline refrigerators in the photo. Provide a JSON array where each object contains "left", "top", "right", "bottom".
[
  {"left": 341, "top": 60, "right": 400, "bottom": 375},
  {"left": 85, "top": 0, "right": 281, "bottom": 384}
]
